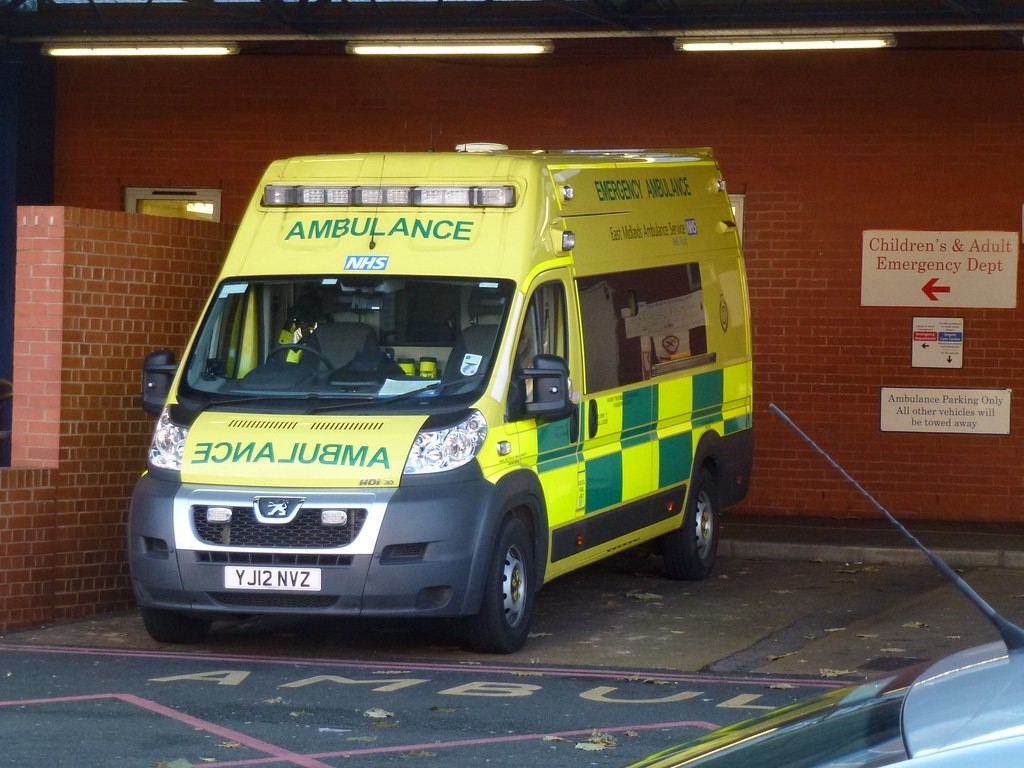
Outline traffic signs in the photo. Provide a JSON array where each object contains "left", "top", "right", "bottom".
[
  {"left": 912, "top": 317, "right": 963, "bottom": 369},
  {"left": 859, "top": 229, "right": 1017, "bottom": 308}
]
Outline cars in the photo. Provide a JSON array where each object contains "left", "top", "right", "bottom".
[{"left": 620, "top": 628, "right": 1024, "bottom": 768}]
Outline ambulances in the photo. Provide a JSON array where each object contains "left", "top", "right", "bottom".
[{"left": 125, "top": 139, "right": 755, "bottom": 655}]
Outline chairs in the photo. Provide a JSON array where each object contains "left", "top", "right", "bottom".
[
  {"left": 300, "top": 323, "right": 379, "bottom": 384},
  {"left": 438, "top": 324, "right": 528, "bottom": 408}
]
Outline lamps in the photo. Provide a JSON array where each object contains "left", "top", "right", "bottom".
[
  {"left": 344, "top": 39, "right": 558, "bottom": 56},
  {"left": 673, "top": 33, "right": 900, "bottom": 52},
  {"left": 40, "top": 41, "right": 238, "bottom": 58}
]
